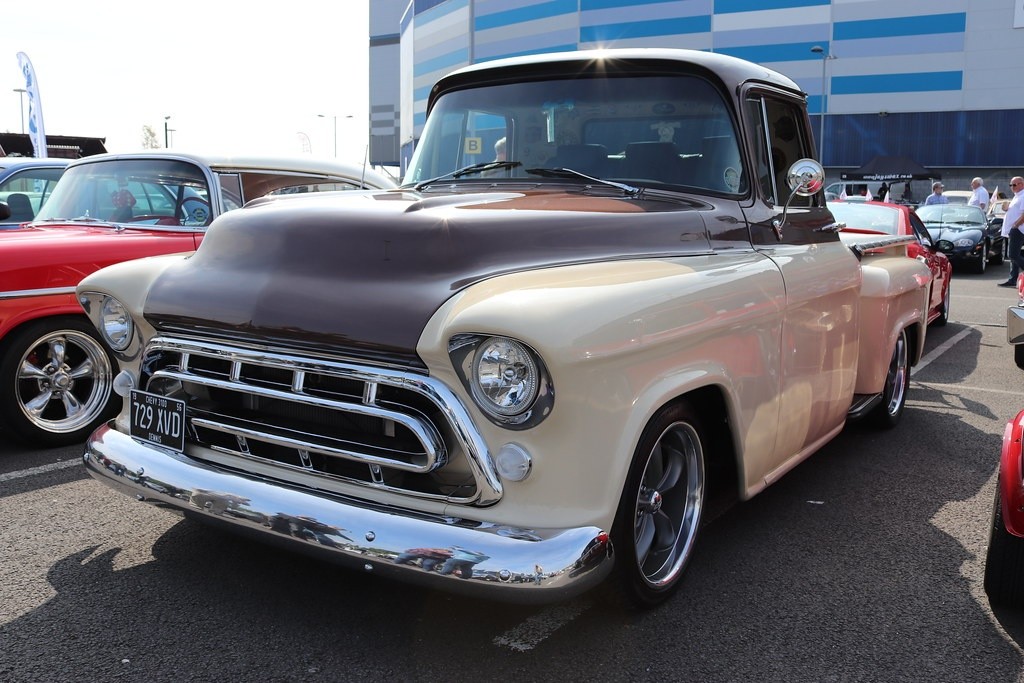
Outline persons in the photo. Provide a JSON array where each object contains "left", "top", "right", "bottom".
[
  {"left": 968, "top": 177, "right": 989, "bottom": 214},
  {"left": 997, "top": 176, "right": 1024, "bottom": 287},
  {"left": 925, "top": 182, "right": 949, "bottom": 206},
  {"left": 481, "top": 136, "right": 507, "bottom": 178},
  {"left": 878, "top": 182, "right": 889, "bottom": 202},
  {"left": 860, "top": 188, "right": 866, "bottom": 196},
  {"left": 901, "top": 183, "right": 913, "bottom": 202}
]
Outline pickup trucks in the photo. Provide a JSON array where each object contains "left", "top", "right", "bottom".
[{"left": 77, "top": 45, "right": 933, "bottom": 619}]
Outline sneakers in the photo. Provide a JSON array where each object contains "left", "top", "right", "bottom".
[{"left": 997, "top": 278, "right": 1016, "bottom": 287}]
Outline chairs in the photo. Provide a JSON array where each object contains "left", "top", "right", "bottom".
[
  {"left": 110, "top": 206, "right": 132, "bottom": 223},
  {"left": 0, "top": 195, "right": 34, "bottom": 220},
  {"left": 563, "top": 134, "right": 742, "bottom": 192}
]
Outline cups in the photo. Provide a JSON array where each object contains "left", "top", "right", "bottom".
[{"left": 1002, "top": 201, "right": 1009, "bottom": 211}]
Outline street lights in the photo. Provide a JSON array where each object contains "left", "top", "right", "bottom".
[
  {"left": 318, "top": 115, "right": 353, "bottom": 158},
  {"left": 168, "top": 130, "right": 176, "bottom": 147},
  {"left": 810, "top": 46, "right": 838, "bottom": 169}
]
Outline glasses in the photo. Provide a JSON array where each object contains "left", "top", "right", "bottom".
[
  {"left": 1009, "top": 182, "right": 1021, "bottom": 187},
  {"left": 940, "top": 187, "right": 944, "bottom": 189}
]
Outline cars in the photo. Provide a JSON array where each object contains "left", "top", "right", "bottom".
[
  {"left": 982, "top": 272, "right": 1024, "bottom": 590},
  {"left": 986, "top": 200, "right": 1011, "bottom": 219},
  {"left": 925, "top": 190, "right": 974, "bottom": 205},
  {"left": 0, "top": 157, "right": 209, "bottom": 227},
  {"left": 915, "top": 204, "right": 1008, "bottom": 274},
  {"left": 825, "top": 201, "right": 951, "bottom": 326},
  {"left": 0, "top": 153, "right": 399, "bottom": 447}
]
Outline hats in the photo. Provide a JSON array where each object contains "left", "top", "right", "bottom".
[{"left": 932, "top": 182, "right": 944, "bottom": 187}]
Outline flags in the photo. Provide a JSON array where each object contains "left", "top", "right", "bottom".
[{"left": 16, "top": 52, "right": 48, "bottom": 157}]
